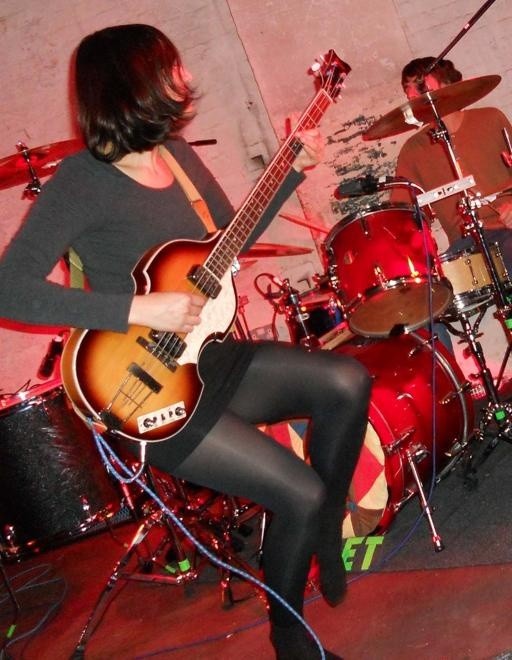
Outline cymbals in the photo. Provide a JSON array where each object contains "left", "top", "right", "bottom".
[
  {"left": 238, "top": 244, "right": 316, "bottom": 258},
  {"left": 362, "top": 74, "right": 502, "bottom": 143},
  {"left": 0, "top": 136, "right": 83, "bottom": 192}
]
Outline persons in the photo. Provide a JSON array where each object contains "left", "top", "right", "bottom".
[
  {"left": 0, "top": 24, "right": 371, "bottom": 660},
  {"left": 390, "top": 57, "right": 512, "bottom": 281}
]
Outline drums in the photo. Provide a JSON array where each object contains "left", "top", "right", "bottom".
[
  {"left": 0, "top": 381, "right": 146, "bottom": 553},
  {"left": 438, "top": 239, "right": 512, "bottom": 323},
  {"left": 320, "top": 200, "right": 451, "bottom": 341},
  {"left": 281, "top": 288, "right": 344, "bottom": 347},
  {"left": 260, "top": 329, "right": 476, "bottom": 538}
]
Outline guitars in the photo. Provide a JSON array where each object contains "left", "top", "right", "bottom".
[{"left": 60, "top": 48, "right": 353, "bottom": 443}]
